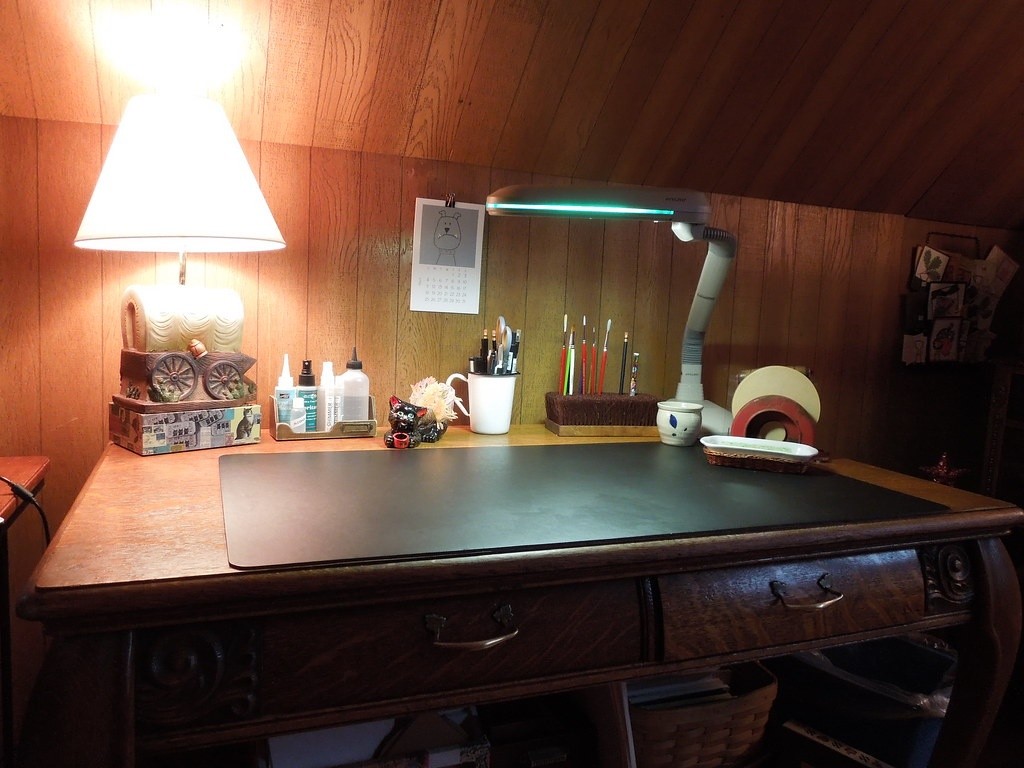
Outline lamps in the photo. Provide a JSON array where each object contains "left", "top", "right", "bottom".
[
  {"left": 72, "top": 90, "right": 289, "bottom": 455},
  {"left": 484, "top": 184, "right": 742, "bottom": 433}
]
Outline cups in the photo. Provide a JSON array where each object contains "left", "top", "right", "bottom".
[
  {"left": 656, "top": 401, "right": 704, "bottom": 447},
  {"left": 446, "top": 371, "right": 521, "bottom": 435}
]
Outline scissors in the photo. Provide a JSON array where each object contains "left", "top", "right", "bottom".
[{"left": 495, "top": 315, "right": 512, "bottom": 373}]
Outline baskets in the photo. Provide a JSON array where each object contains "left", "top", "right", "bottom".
[{"left": 590, "top": 661, "right": 777, "bottom": 768}]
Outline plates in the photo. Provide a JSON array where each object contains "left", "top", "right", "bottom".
[
  {"left": 699, "top": 434, "right": 819, "bottom": 462},
  {"left": 732, "top": 365, "right": 821, "bottom": 424}
]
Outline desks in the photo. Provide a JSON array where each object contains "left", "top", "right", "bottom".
[
  {"left": 0, "top": 455, "right": 51, "bottom": 528},
  {"left": 26, "top": 423, "right": 1024, "bottom": 768}
]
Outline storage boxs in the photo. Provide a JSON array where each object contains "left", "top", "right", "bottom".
[{"left": 106, "top": 403, "right": 259, "bottom": 458}]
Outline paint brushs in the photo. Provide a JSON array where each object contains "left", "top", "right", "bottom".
[{"left": 558, "top": 312, "right": 640, "bottom": 397}]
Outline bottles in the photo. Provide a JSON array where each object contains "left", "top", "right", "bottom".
[{"left": 274, "top": 347, "right": 370, "bottom": 434}]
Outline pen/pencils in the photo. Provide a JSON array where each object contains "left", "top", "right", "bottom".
[{"left": 468, "top": 326, "right": 522, "bottom": 375}]
